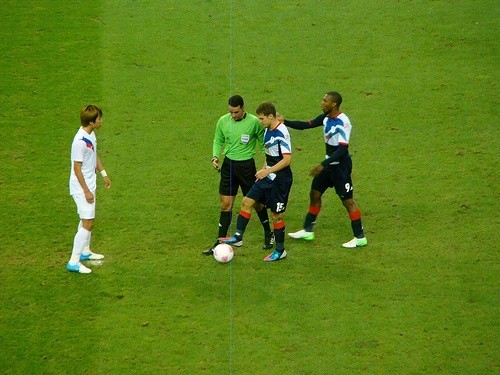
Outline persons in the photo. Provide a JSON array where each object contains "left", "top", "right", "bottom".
[
  {"left": 276, "top": 92, "right": 368, "bottom": 248},
  {"left": 218, "top": 103, "right": 293, "bottom": 262},
  {"left": 66, "top": 104, "right": 111, "bottom": 273},
  {"left": 202, "top": 95, "right": 275, "bottom": 256}
]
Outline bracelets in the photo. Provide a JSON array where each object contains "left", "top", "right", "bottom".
[{"left": 100, "top": 170, "right": 107, "bottom": 177}]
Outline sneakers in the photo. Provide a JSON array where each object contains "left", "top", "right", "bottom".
[
  {"left": 81, "top": 252, "right": 104, "bottom": 259},
  {"left": 66, "top": 261, "right": 91, "bottom": 273},
  {"left": 343, "top": 236, "right": 367, "bottom": 247},
  {"left": 202, "top": 240, "right": 221, "bottom": 254},
  {"left": 217, "top": 231, "right": 242, "bottom": 247},
  {"left": 263, "top": 232, "right": 275, "bottom": 248},
  {"left": 263, "top": 249, "right": 287, "bottom": 261},
  {"left": 287, "top": 229, "right": 314, "bottom": 240}
]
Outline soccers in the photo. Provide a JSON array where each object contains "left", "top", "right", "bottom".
[{"left": 213, "top": 243, "right": 234, "bottom": 263}]
